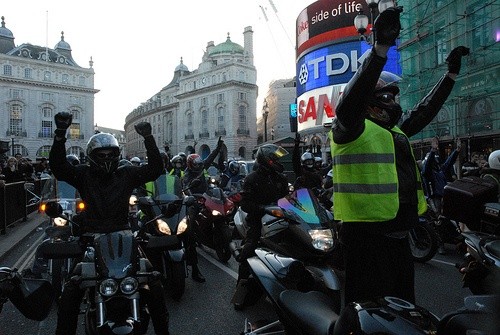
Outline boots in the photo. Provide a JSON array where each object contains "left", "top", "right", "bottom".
[{"left": 232, "top": 279, "right": 248, "bottom": 306}]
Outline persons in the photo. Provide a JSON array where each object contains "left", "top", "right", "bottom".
[
  {"left": 0, "top": 153, "right": 51, "bottom": 182},
  {"left": 223, "top": 160, "right": 246, "bottom": 190},
  {"left": 329, "top": 6, "right": 471, "bottom": 303},
  {"left": 292, "top": 132, "right": 330, "bottom": 200},
  {"left": 231, "top": 144, "right": 292, "bottom": 310},
  {"left": 422, "top": 138, "right": 461, "bottom": 200},
  {"left": 47, "top": 111, "right": 170, "bottom": 335},
  {"left": 462, "top": 149, "right": 500, "bottom": 185},
  {"left": 180, "top": 136, "right": 225, "bottom": 228},
  {"left": 120, "top": 151, "right": 205, "bottom": 284}
]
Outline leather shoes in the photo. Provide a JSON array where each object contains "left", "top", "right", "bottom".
[{"left": 192, "top": 263, "right": 207, "bottom": 283}]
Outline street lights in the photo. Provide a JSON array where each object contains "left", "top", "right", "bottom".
[
  {"left": 313, "top": 135, "right": 318, "bottom": 157},
  {"left": 262, "top": 102, "right": 270, "bottom": 142},
  {"left": 93, "top": 123, "right": 100, "bottom": 134},
  {"left": 10, "top": 133, "right": 16, "bottom": 157},
  {"left": 353, "top": 0, "right": 395, "bottom": 53}
]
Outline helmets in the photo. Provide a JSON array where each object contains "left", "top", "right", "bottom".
[
  {"left": 66, "top": 154, "right": 80, "bottom": 167},
  {"left": 171, "top": 156, "right": 183, "bottom": 170},
  {"left": 131, "top": 156, "right": 142, "bottom": 167},
  {"left": 301, "top": 152, "right": 316, "bottom": 169},
  {"left": 425, "top": 152, "right": 439, "bottom": 164},
  {"left": 159, "top": 149, "right": 168, "bottom": 172},
  {"left": 229, "top": 160, "right": 238, "bottom": 174},
  {"left": 256, "top": 144, "right": 292, "bottom": 178},
  {"left": 314, "top": 157, "right": 323, "bottom": 169},
  {"left": 86, "top": 132, "right": 120, "bottom": 178},
  {"left": 367, "top": 70, "right": 403, "bottom": 130},
  {"left": 488, "top": 150, "right": 500, "bottom": 170},
  {"left": 187, "top": 154, "right": 205, "bottom": 173}
]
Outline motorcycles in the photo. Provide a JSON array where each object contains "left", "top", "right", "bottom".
[
  {"left": 35, "top": 199, "right": 184, "bottom": 335},
  {"left": 229, "top": 187, "right": 342, "bottom": 312},
  {"left": 184, "top": 186, "right": 241, "bottom": 264},
  {"left": 442, "top": 177, "right": 500, "bottom": 294},
  {"left": 242, "top": 246, "right": 500, "bottom": 334},
  {"left": 408, "top": 221, "right": 438, "bottom": 262},
  {"left": 135, "top": 175, "right": 202, "bottom": 302}
]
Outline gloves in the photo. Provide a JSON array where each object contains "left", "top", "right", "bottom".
[
  {"left": 54, "top": 111, "right": 73, "bottom": 136},
  {"left": 217, "top": 136, "right": 224, "bottom": 148},
  {"left": 373, "top": 6, "right": 404, "bottom": 47},
  {"left": 134, "top": 121, "right": 152, "bottom": 137},
  {"left": 444, "top": 45, "right": 471, "bottom": 75}
]
